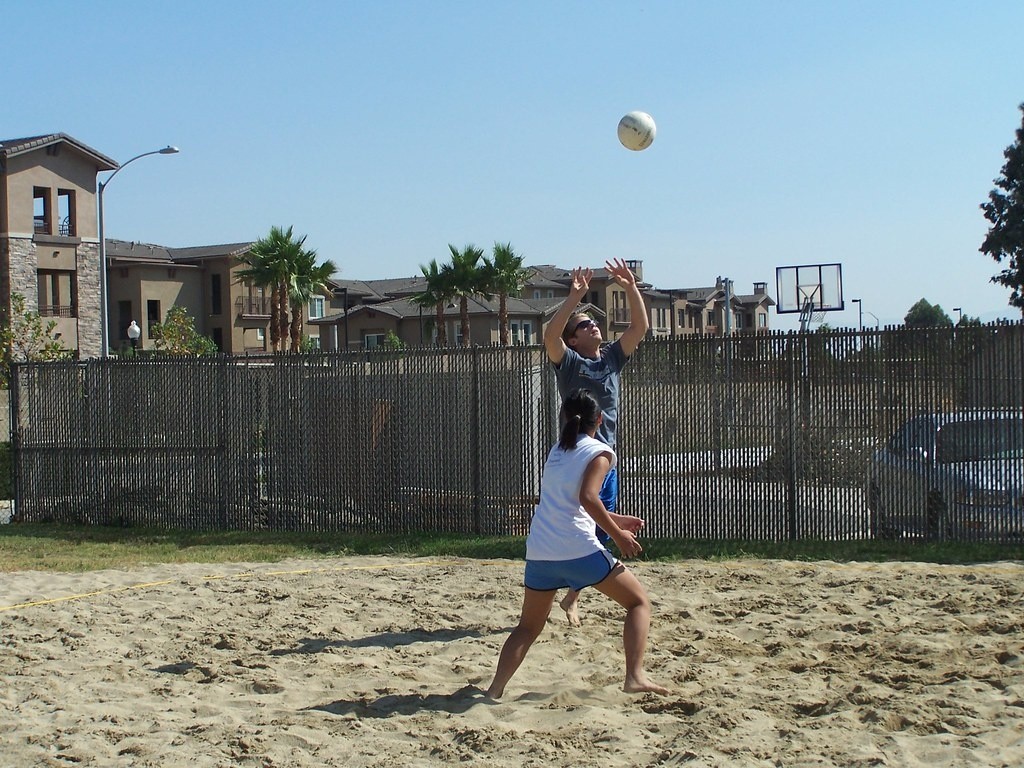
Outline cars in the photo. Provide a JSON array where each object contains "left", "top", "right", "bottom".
[{"left": 868, "top": 409, "right": 1024, "bottom": 549}]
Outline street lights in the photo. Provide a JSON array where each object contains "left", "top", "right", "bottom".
[
  {"left": 851, "top": 299, "right": 862, "bottom": 359},
  {"left": 98, "top": 146, "right": 180, "bottom": 415},
  {"left": 860, "top": 311, "right": 880, "bottom": 333},
  {"left": 127, "top": 320, "right": 140, "bottom": 368},
  {"left": 953, "top": 308, "right": 961, "bottom": 322}
]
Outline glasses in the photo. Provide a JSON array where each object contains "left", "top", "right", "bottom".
[{"left": 572, "top": 319, "right": 596, "bottom": 338}]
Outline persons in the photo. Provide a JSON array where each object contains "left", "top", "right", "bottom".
[
  {"left": 544, "top": 258, "right": 649, "bottom": 627},
  {"left": 484, "top": 388, "right": 672, "bottom": 700}
]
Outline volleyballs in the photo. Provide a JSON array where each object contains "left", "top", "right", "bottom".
[{"left": 617, "top": 110, "right": 658, "bottom": 151}]
